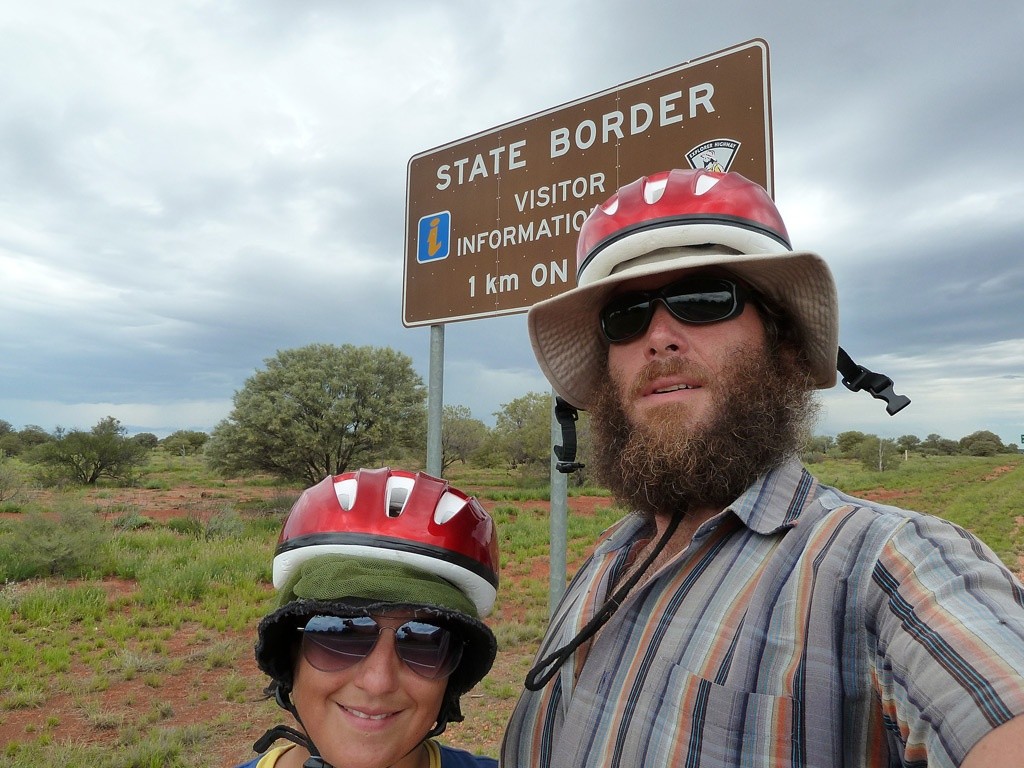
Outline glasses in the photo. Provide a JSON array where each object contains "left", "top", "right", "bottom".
[
  {"left": 294, "top": 613, "right": 469, "bottom": 680},
  {"left": 599, "top": 270, "right": 775, "bottom": 343}
]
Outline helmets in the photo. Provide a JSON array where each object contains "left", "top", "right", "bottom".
[
  {"left": 274, "top": 465, "right": 498, "bottom": 617},
  {"left": 576, "top": 167, "right": 796, "bottom": 284}
]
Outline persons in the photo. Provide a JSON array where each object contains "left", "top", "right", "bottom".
[
  {"left": 237, "top": 469, "right": 501, "bottom": 768},
  {"left": 500, "top": 168, "right": 1024, "bottom": 768}
]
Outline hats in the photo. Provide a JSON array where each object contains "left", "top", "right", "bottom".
[
  {"left": 255, "top": 559, "right": 498, "bottom": 725},
  {"left": 527, "top": 251, "right": 839, "bottom": 411}
]
